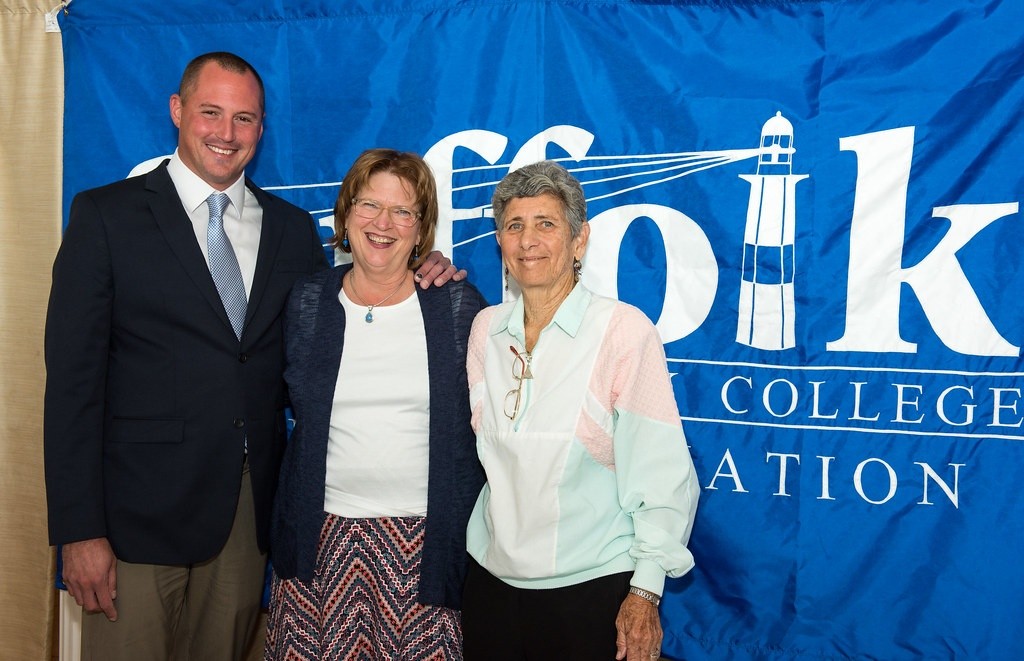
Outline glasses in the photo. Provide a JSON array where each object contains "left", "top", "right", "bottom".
[
  {"left": 351, "top": 197, "right": 423, "bottom": 228},
  {"left": 503, "top": 344, "right": 534, "bottom": 420}
]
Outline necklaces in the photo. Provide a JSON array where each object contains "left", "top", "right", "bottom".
[{"left": 348, "top": 268, "right": 404, "bottom": 323}]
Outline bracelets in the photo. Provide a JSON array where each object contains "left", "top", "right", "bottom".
[{"left": 629, "top": 589, "right": 660, "bottom": 605}]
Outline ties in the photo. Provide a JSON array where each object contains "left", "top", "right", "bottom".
[{"left": 202, "top": 191, "right": 249, "bottom": 343}]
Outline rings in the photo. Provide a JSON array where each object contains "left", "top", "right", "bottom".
[{"left": 650, "top": 654, "right": 658, "bottom": 657}]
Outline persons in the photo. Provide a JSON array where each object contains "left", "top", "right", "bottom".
[
  {"left": 44, "top": 52, "right": 470, "bottom": 661},
  {"left": 262, "top": 149, "right": 486, "bottom": 661},
  {"left": 465, "top": 157, "right": 702, "bottom": 661}
]
